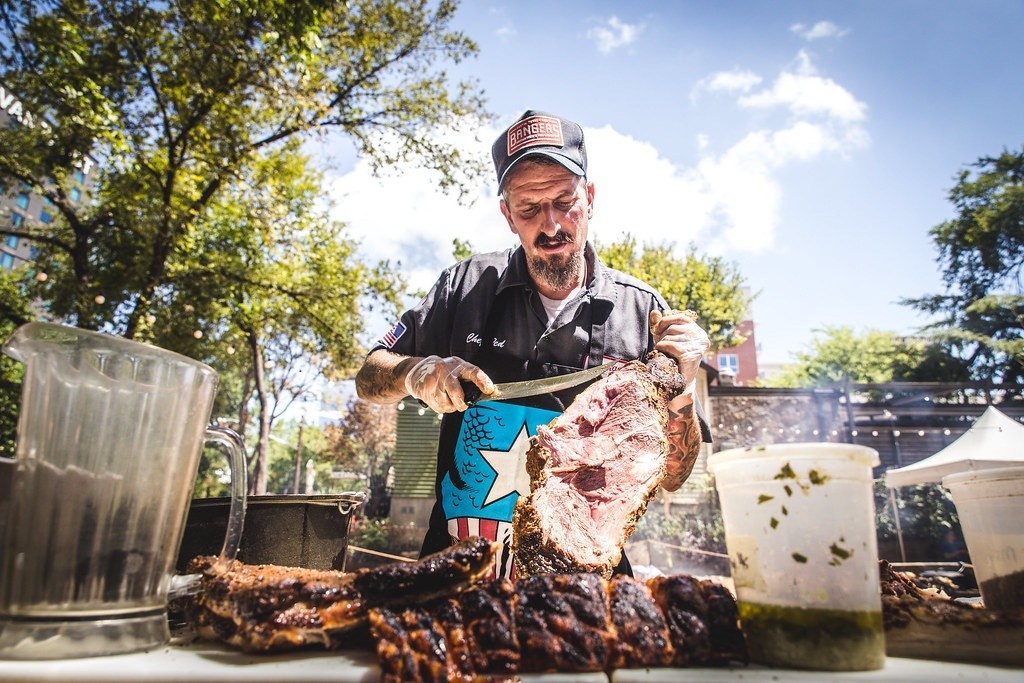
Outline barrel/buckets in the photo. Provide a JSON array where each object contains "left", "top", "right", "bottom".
[
  {"left": 940, "top": 466, "right": 1024, "bottom": 624},
  {"left": 167, "top": 493, "right": 360, "bottom": 624},
  {"left": 706, "top": 443, "right": 884, "bottom": 670}
]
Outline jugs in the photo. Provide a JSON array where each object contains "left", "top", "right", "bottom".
[{"left": 0, "top": 321, "right": 247, "bottom": 661}]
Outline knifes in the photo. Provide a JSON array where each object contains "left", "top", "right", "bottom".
[{"left": 420, "top": 358, "right": 619, "bottom": 409}]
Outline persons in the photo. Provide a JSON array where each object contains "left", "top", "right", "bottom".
[{"left": 356, "top": 110, "right": 707, "bottom": 574}]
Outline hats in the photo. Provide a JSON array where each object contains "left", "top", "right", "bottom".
[{"left": 491, "top": 109, "right": 588, "bottom": 197}]
